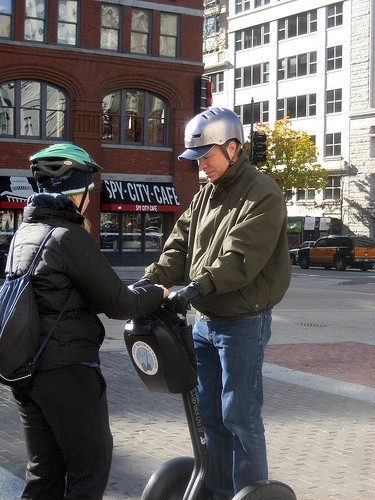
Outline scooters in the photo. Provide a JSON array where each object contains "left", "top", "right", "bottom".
[{"left": 124, "top": 308, "right": 297, "bottom": 500}]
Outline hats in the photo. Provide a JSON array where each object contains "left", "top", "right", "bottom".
[
  {"left": 178, "top": 145, "right": 215, "bottom": 161},
  {"left": 37, "top": 170, "right": 94, "bottom": 195}
]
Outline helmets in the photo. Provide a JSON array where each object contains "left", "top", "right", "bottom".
[
  {"left": 29, "top": 143, "right": 103, "bottom": 181},
  {"left": 184, "top": 109, "right": 243, "bottom": 149}
]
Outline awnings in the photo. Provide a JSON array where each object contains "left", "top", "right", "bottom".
[
  {"left": 0, "top": 175, "right": 39, "bottom": 210},
  {"left": 101, "top": 179, "right": 181, "bottom": 212}
]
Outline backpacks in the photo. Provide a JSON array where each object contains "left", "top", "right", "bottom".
[{"left": 0, "top": 226, "right": 76, "bottom": 386}]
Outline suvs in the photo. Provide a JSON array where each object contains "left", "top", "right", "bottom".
[
  {"left": 297, "top": 234, "right": 375, "bottom": 271},
  {"left": 289, "top": 240, "right": 317, "bottom": 266}
]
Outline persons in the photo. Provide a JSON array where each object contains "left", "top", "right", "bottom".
[
  {"left": 0, "top": 143, "right": 170, "bottom": 500},
  {"left": 129, "top": 107, "right": 292, "bottom": 500}
]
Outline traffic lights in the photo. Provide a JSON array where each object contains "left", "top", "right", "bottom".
[{"left": 249, "top": 133, "right": 267, "bottom": 165}]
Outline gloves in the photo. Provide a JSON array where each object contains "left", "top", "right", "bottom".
[
  {"left": 128, "top": 279, "right": 152, "bottom": 291},
  {"left": 168, "top": 283, "right": 203, "bottom": 317}
]
